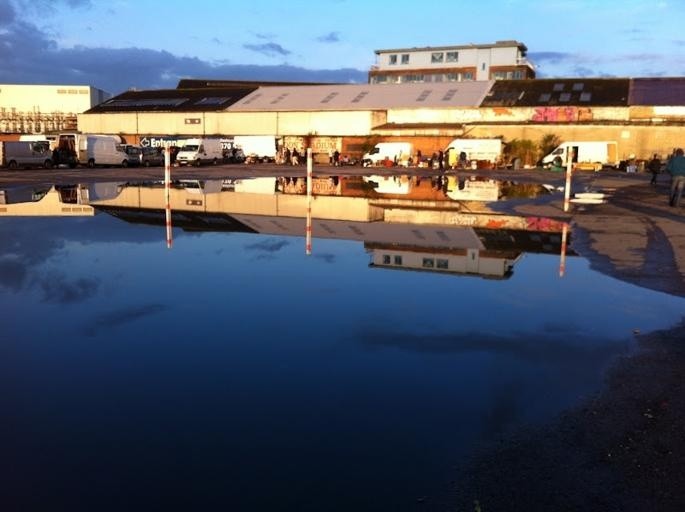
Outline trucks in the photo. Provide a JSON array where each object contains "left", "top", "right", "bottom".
[
  {"left": 1, "top": 140, "right": 54, "bottom": 169},
  {"left": 362, "top": 142, "right": 412, "bottom": 167},
  {"left": 447, "top": 139, "right": 501, "bottom": 170},
  {"left": 176, "top": 138, "right": 224, "bottom": 167},
  {"left": 20, "top": 133, "right": 78, "bottom": 168},
  {"left": 231, "top": 134, "right": 282, "bottom": 163},
  {"left": 540, "top": 138, "right": 608, "bottom": 164},
  {"left": 77, "top": 133, "right": 129, "bottom": 167}
]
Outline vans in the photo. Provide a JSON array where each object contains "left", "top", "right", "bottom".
[{"left": 127, "top": 144, "right": 163, "bottom": 167}]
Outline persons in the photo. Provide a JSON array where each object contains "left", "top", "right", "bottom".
[
  {"left": 508, "top": 156, "right": 522, "bottom": 170},
  {"left": 443, "top": 149, "right": 451, "bottom": 170},
  {"left": 551, "top": 157, "right": 564, "bottom": 168},
  {"left": 283, "top": 147, "right": 294, "bottom": 167},
  {"left": 442, "top": 176, "right": 449, "bottom": 197},
  {"left": 438, "top": 148, "right": 444, "bottom": 170},
  {"left": 333, "top": 149, "right": 341, "bottom": 166},
  {"left": 649, "top": 153, "right": 662, "bottom": 185},
  {"left": 664, "top": 148, "right": 685, "bottom": 208},
  {"left": 436, "top": 175, "right": 443, "bottom": 191},
  {"left": 291, "top": 149, "right": 300, "bottom": 166},
  {"left": 328, "top": 148, "right": 334, "bottom": 166},
  {"left": 341, "top": 155, "right": 349, "bottom": 166}
]
